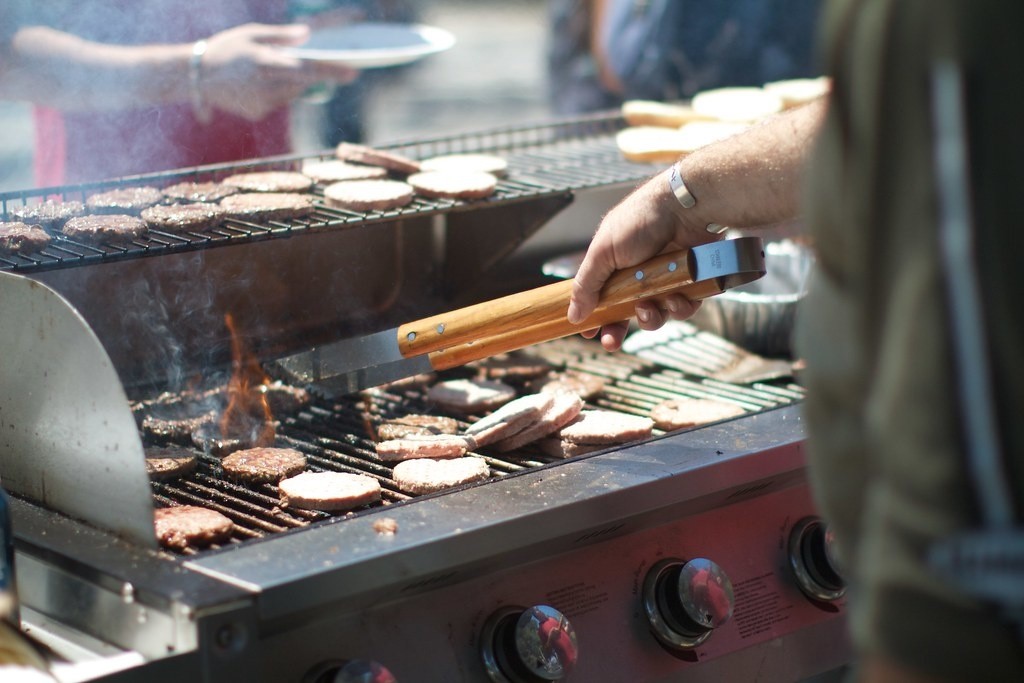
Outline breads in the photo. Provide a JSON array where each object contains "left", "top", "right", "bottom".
[{"left": 615, "top": 77, "right": 828, "bottom": 163}]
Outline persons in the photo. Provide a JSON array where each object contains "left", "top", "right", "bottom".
[
  {"left": 0, "top": 0, "right": 456, "bottom": 204},
  {"left": 548, "top": 0, "right": 826, "bottom": 139},
  {"left": 567, "top": 0, "right": 1024, "bottom": 683}
]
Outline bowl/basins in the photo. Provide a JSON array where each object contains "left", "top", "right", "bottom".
[{"left": 683, "top": 245, "right": 804, "bottom": 351}]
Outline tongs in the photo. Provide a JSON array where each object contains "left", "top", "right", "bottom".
[{"left": 263, "top": 237, "right": 767, "bottom": 400}]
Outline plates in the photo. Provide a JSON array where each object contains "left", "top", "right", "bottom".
[{"left": 272, "top": 23, "right": 455, "bottom": 67}]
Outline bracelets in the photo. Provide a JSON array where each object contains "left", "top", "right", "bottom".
[
  {"left": 189, "top": 41, "right": 213, "bottom": 126},
  {"left": 667, "top": 161, "right": 729, "bottom": 234}
]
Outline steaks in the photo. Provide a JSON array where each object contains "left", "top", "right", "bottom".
[
  {"left": 0, "top": 141, "right": 508, "bottom": 253},
  {"left": 140, "top": 346, "right": 748, "bottom": 547}
]
